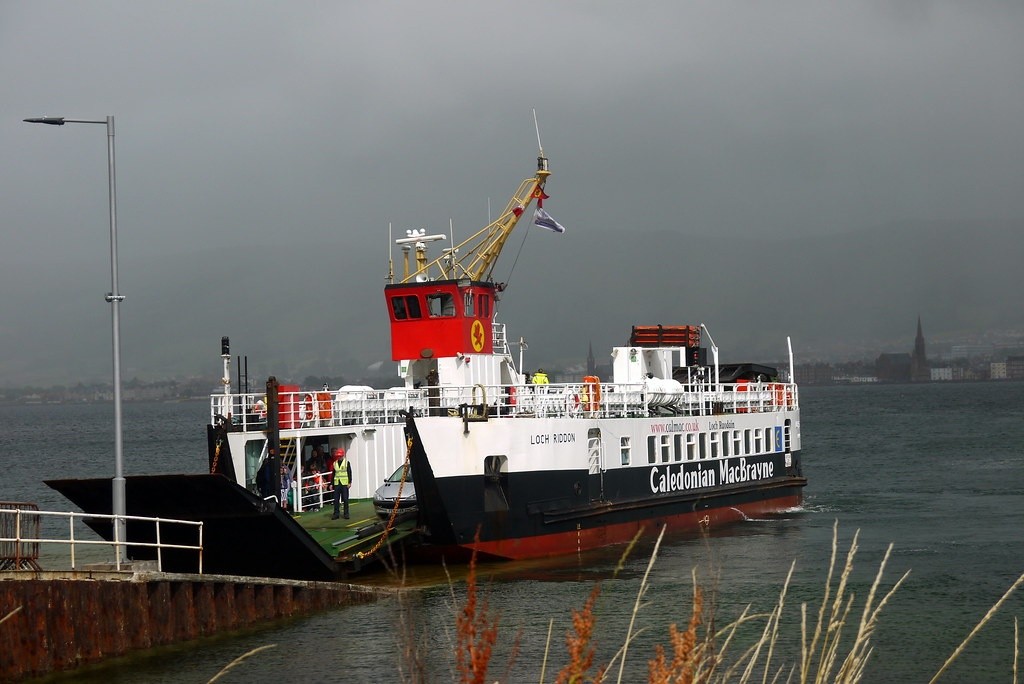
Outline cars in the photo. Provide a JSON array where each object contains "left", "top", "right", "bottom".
[{"left": 373, "top": 462, "right": 418, "bottom": 519}]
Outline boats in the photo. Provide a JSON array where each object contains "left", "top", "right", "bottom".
[{"left": 42, "top": 157, "right": 809, "bottom": 581}]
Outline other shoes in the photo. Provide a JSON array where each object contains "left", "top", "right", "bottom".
[
  {"left": 345, "top": 516, "right": 349, "bottom": 519},
  {"left": 302, "top": 509, "right": 307, "bottom": 512},
  {"left": 314, "top": 508, "right": 319, "bottom": 512},
  {"left": 331, "top": 516, "right": 339, "bottom": 520}
]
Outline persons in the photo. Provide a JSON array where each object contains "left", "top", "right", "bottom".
[
  {"left": 280, "top": 467, "right": 290, "bottom": 509},
  {"left": 325, "top": 447, "right": 338, "bottom": 505},
  {"left": 756, "top": 374, "right": 767, "bottom": 390},
  {"left": 317, "top": 446, "right": 332, "bottom": 472},
  {"left": 256, "top": 449, "right": 276, "bottom": 497},
  {"left": 306, "top": 450, "right": 321, "bottom": 473},
  {"left": 329, "top": 449, "right": 352, "bottom": 520}
]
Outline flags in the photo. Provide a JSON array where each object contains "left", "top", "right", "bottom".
[{"left": 534, "top": 208, "right": 565, "bottom": 233}]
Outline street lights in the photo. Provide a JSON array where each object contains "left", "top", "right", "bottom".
[{"left": 21, "top": 117, "right": 130, "bottom": 566}]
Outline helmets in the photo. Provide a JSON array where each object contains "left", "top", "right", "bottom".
[{"left": 335, "top": 449, "right": 344, "bottom": 456}]
